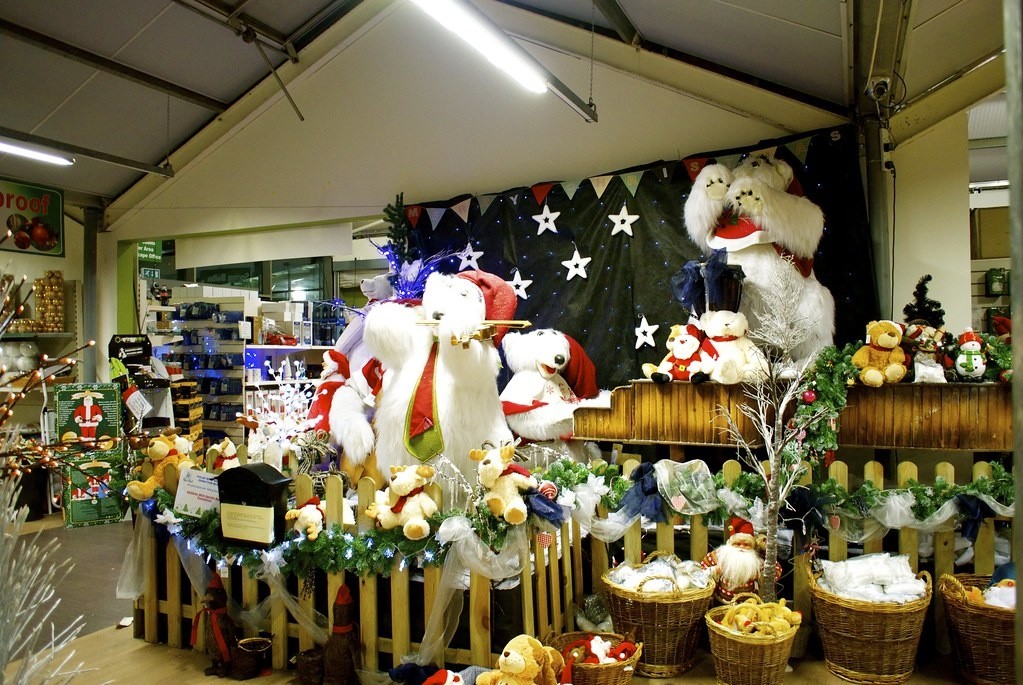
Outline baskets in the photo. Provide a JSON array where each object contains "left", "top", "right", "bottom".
[
  {"left": 705, "top": 592, "right": 802, "bottom": 685},
  {"left": 802, "top": 552, "right": 932, "bottom": 685},
  {"left": 541, "top": 630, "right": 643, "bottom": 685},
  {"left": 936, "top": 572, "right": 1016, "bottom": 685},
  {"left": 600, "top": 549, "right": 716, "bottom": 678}
]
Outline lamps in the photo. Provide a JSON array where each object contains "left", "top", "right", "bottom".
[
  {"left": 0, "top": 94, "right": 174, "bottom": 178},
  {"left": 413, "top": 0, "right": 599, "bottom": 126}
]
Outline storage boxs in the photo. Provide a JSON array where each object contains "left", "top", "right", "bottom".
[{"left": 171, "top": 286, "right": 351, "bottom": 346}]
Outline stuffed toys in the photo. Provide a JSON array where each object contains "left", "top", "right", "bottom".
[
  {"left": 646, "top": 149, "right": 986, "bottom": 387},
  {"left": 126, "top": 259, "right": 636, "bottom": 685}
]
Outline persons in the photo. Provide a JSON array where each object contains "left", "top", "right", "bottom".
[
  {"left": 703, "top": 517, "right": 782, "bottom": 608},
  {"left": 72, "top": 395, "right": 104, "bottom": 439}
]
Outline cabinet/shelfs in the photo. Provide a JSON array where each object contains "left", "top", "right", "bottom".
[
  {"left": 0, "top": 280, "right": 84, "bottom": 435},
  {"left": 147, "top": 304, "right": 338, "bottom": 466}
]
